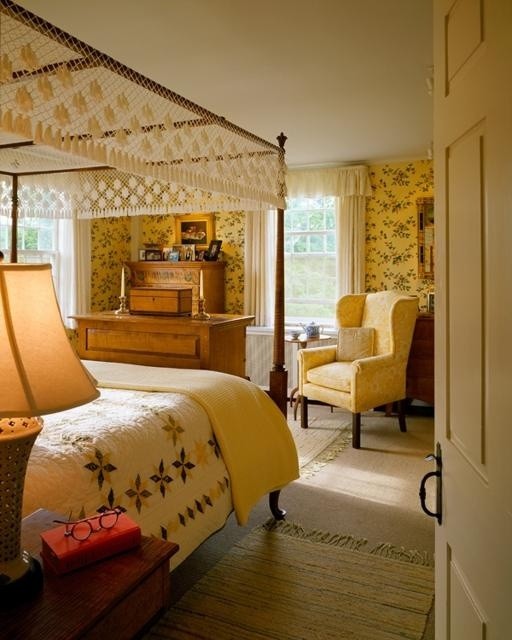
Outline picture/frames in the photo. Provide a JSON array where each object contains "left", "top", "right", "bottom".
[{"left": 138, "top": 214, "right": 223, "bottom": 261}]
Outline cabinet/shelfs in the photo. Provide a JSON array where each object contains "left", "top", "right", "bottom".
[
  {"left": 67, "top": 310, "right": 255, "bottom": 378},
  {"left": 396, "top": 312, "right": 434, "bottom": 431},
  {"left": 427, "top": 292, "right": 434, "bottom": 314},
  {"left": 125, "top": 261, "right": 227, "bottom": 313}
]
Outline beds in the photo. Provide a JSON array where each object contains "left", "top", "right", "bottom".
[{"left": 0, "top": 0, "right": 301, "bottom": 574}]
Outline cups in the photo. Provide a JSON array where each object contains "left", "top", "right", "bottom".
[{"left": 290, "top": 331, "right": 300, "bottom": 340}]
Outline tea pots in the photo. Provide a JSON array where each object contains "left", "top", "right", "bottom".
[{"left": 300, "top": 321, "right": 324, "bottom": 339}]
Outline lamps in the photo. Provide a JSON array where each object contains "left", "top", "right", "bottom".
[{"left": 0, "top": 252, "right": 100, "bottom": 609}]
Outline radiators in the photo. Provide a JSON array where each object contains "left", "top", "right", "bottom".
[{"left": 246, "top": 326, "right": 305, "bottom": 400}]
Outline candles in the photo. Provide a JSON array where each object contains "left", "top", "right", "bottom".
[
  {"left": 121, "top": 266, "right": 125, "bottom": 298},
  {"left": 200, "top": 269, "right": 203, "bottom": 299}
]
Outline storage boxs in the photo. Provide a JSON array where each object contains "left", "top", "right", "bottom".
[{"left": 128, "top": 287, "right": 192, "bottom": 318}]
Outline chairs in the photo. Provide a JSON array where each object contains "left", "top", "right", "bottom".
[{"left": 296, "top": 292, "right": 420, "bottom": 449}]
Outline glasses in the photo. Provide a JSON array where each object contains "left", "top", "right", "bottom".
[{"left": 53, "top": 509, "right": 121, "bottom": 541}]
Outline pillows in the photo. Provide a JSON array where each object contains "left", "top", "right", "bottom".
[{"left": 336, "top": 327, "right": 376, "bottom": 362}]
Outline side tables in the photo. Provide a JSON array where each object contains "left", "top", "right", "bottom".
[{"left": 284, "top": 334, "right": 333, "bottom": 421}]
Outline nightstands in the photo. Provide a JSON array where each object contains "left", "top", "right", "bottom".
[{"left": 0, "top": 507, "right": 180, "bottom": 640}]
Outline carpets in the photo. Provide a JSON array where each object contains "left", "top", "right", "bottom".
[
  {"left": 285, "top": 401, "right": 363, "bottom": 480},
  {"left": 143, "top": 517, "right": 435, "bottom": 640}
]
